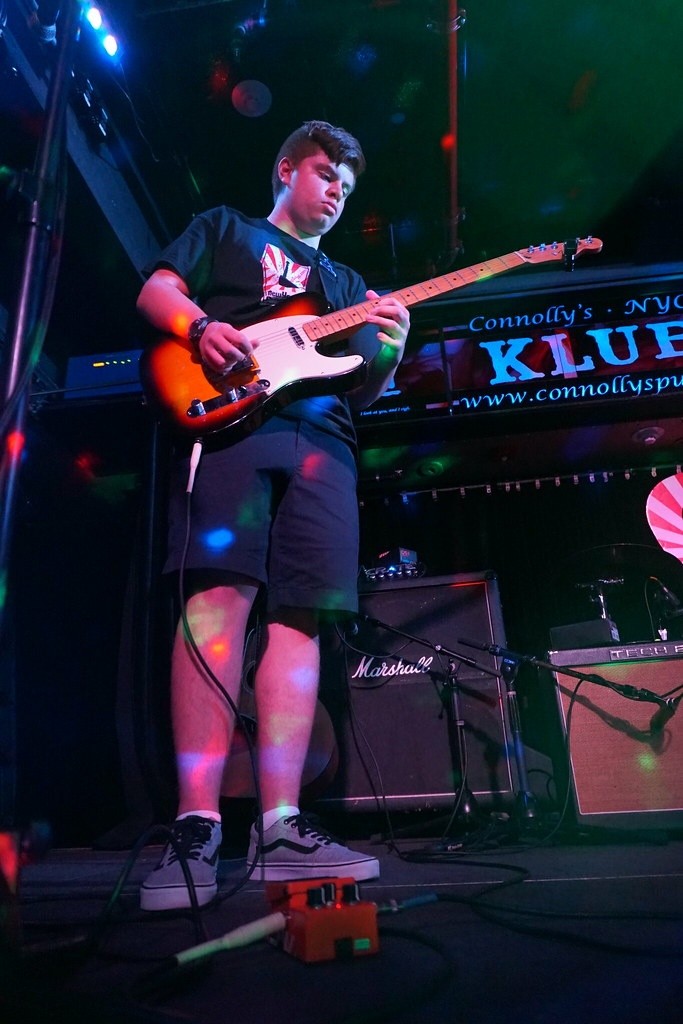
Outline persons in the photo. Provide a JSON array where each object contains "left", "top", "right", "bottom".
[{"left": 133, "top": 119, "right": 411, "bottom": 910}]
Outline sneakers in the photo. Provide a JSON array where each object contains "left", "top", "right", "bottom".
[
  {"left": 138, "top": 816, "right": 224, "bottom": 912},
  {"left": 245, "top": 812, "right": 381, "bottom": 885}
]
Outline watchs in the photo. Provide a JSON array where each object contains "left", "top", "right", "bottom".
[{"left": 187, "top": 316, "right": 220, "bottom": 353}]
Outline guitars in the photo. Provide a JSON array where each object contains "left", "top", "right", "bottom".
[{"left": 139, "top": 236, "right": 604, "bottom": 454}]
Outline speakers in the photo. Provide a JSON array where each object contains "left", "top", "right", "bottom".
[
  {"left": 296, "top": 565, "right": 536, "bottom": 817},
  {"left": 543, "top": 639, "right": 683, "bottom": 829}
]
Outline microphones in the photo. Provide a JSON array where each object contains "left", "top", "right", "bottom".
[
  {"left": 650, "top": 697, "right": 681, "bottom": 729},
  {"left": 168, "top": 910, "right": 287, "bottom": 966}
]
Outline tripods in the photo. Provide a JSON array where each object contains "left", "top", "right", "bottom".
[
  {"left": 362, "top": 616, "right": 665, "bottom": 849},
  {"left": 84, "top": 408, "right": 213, "bottom": 945}
]
